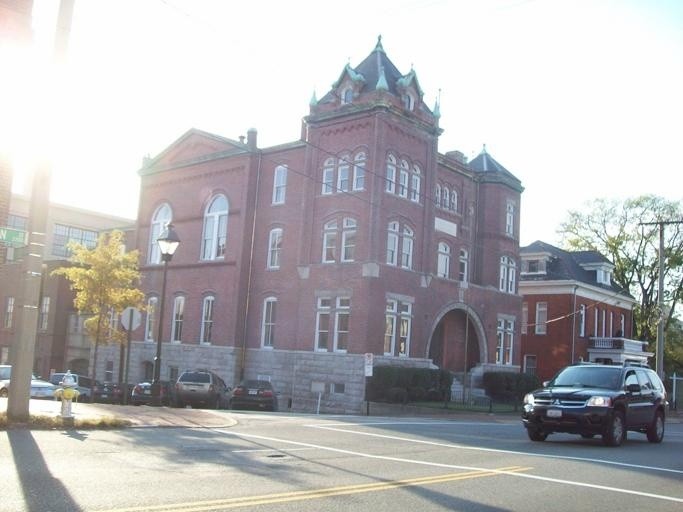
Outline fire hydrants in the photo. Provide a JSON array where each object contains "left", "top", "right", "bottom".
[{"left": 53, "top": 369, "right": 82, "bottom": 419}]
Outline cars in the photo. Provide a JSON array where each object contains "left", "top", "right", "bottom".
[
  {"left": 229, "top": 377, "right": 282, "bottom": 413},
  {"left": 125, "top": 377, "right": 189, "bottom": 409},
  {"left": 0, "top": 363, "right": 58, "bottom": 400},
  {"left": 86, "top": 381, "right": 141, "bottom": 408}
]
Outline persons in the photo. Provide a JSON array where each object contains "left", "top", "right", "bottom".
[{"left": 608, "top": 375, "right": 618, "bottom": 389}]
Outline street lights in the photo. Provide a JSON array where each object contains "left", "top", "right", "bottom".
[{"left": 141, "top": 219, "right": 182, "bottom": 410}]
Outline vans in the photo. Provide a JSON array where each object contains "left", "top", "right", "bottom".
[{"left": 46, "top": 371, "right": 103, "bottom": 405}]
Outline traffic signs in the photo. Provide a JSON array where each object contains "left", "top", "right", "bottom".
[{"left": 0, "top": 226, "right": 28, "bottom": 244}]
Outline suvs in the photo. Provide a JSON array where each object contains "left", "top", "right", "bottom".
[
  {"left": 516, "top": 360, "right": 670, "bottom": 448},
  {"left": 167, "top": 368, "right": 234, "bottom": 411}
]
